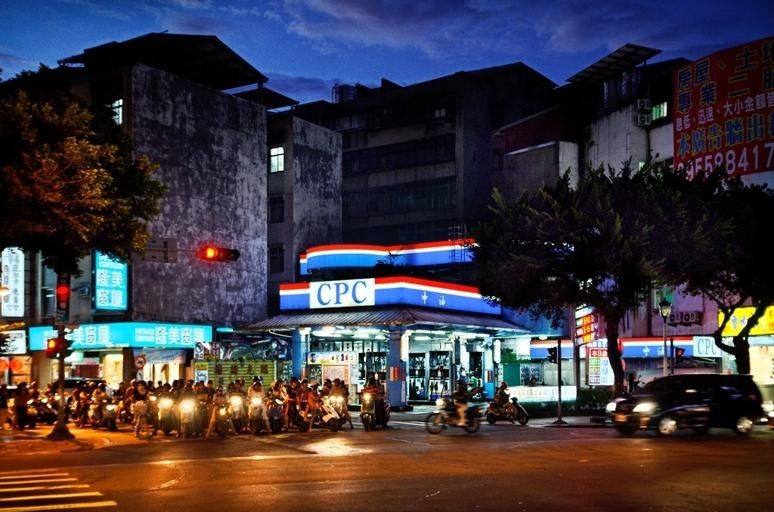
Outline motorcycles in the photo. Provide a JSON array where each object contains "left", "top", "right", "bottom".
[
  {"left": 359, "top": 386, "right": 390, "bottom": 432},
  {"left": 425, "top": 396, "right": 486, "bottom": 434},
  {"left": 7, "top": 390, "right": 347, "bottom": 439},
  {"left": 485, "top": 397, "right": 529, "bottom": 426}
]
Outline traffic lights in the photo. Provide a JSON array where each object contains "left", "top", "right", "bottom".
[
  {"left": 673, "top": 345, "right": 687, "bottom": 360},
  {"left": 47, "top": 338, "right": 65, "bottom": 358},
  {"left": 55, "top": 263, "right": 72, "bottom": 327},
  {"left": 197, "top": 244, "right": 241, "bottom": 263}
]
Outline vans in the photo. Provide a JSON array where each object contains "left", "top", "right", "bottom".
[
  {"left": 70, "top": 376, "right": 106, "bottom": 384},
  {"left": 606, "top": 374, "right": 762, "bottom": 439}
]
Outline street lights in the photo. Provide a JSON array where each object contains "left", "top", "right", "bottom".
[{"left": 659, "top": 296, "right": 673, "bottom": 377}]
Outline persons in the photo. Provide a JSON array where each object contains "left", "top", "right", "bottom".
[
  {"left": 627, "top": 372, "right": 641, "bottom": 394},
  {"left": 0, "top": 373, "right": 391, "bottom": 438},
  {"left": 459, "top": 366, "right": 467, "bottom": 380},
  {"left": 447, "top": 377, "right": 473, "bottom": 429},
  {"left": 494, "top": 381, "right": 513, "bottom": 414}
]
